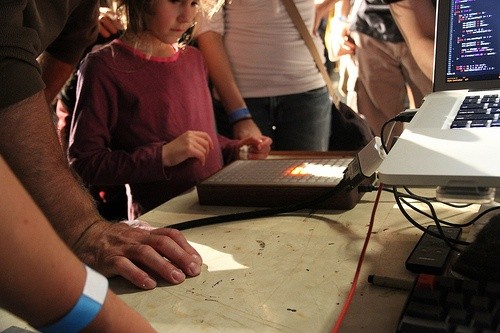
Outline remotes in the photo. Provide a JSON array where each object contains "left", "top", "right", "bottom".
[{"left": 405, "top": 224, "right": 462, "bottom": 275}]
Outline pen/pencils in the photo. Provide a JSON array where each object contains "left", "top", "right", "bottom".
[{"left": 366, "top": 274, "right": 412, "bottom": 289}]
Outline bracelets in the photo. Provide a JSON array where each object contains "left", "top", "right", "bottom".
[
  {"left": 37, "top": 266, "right": 110, "bottom": 333},
  {"left": 228, "top": 106, "right": 252, "bottom": 124}
]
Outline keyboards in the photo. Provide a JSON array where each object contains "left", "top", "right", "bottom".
[{"left": 397, "top": 272, "right": 500, "bottom": 333}]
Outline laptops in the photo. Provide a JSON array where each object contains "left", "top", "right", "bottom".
[{"left": 379, "top": 0, "right": 500, "bottom": 187}]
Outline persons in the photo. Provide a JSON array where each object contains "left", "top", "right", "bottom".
[
  {"left": 196, "top": 0, "right": 341, "bottom": 152},
  {"left": 67, "top": 0, "right": 273, "bottom": 220},
  {"left": 54, "top": 0, "right": 129, "bottom": 154},
  {"left": 0, "top": 0, "right": 203, "bottom": 291},
  {"left": 0, "top": 152, "right": 157, "bottom": 332},
  {"left": 318, "top": 0, "right": 438, "bottom": 152}
]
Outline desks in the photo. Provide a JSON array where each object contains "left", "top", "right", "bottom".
[{"left": 0, "top": 183, "right": 483, "bottom": 333}]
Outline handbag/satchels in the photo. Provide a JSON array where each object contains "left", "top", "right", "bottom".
[{"left": 328, "top": 100, "right": 373, "bottom": 151}]
